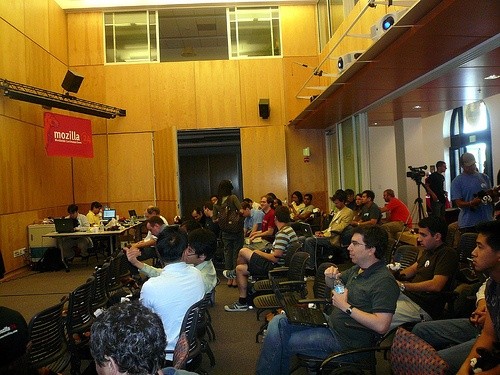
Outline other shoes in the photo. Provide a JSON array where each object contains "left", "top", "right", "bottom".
[
  {"left": 67, "top": 252, "right": 75, "bottom": 261},
  {"left": 81, "top": 249, "right": 89, "bottom": 258},
  {"left": 228, "top": 284, "right": 237, "bottom": 288}
]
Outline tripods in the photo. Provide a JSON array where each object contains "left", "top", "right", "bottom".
[{"left": 391, "top": 181, "right": 428, "bottom": 257}]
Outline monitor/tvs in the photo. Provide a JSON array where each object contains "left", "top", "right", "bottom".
[
  {"left": 102, "top": 209, "right": 116, "bottom": 220},
  {"left": 128, "top": 210, "right": 137, "bottom": 218}
]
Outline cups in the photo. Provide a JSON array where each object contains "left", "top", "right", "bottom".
[
  {"left": 93, "top": 225, "right": 98, "bottom": 232},
  {"left": 244, "top": 237, "right": 250, "bottom": 246}
]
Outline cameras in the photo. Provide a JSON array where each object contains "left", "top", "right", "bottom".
[{"left": 406, "top": 165, "right": 427, "bottom": 182}]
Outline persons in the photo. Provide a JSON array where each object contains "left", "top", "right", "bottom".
[
  {"left": 455, "top": 220, "right": 500, "bottom": 375},
  {"left": 61, "top": 204, "right": 93, "bottom": 264},
  {"left": 89, "top": 300, "right": 201, "bottom": 375},
  {"left": 492, "top": 202, "right": 500, "bottom": 222},
  {"left": 85, "top": 201, "right": 117, "bottom": 258},
  {"left": 121, "top": 161, "right": 449, "bottom": 288},
  {"left": 140, "top": 226, "right": 205, "bottom": 371},
  {"left": 223, "top": 206, "right": 299, "bottom": 313},
  {"left": 380, "top": 215, "right": 459, "bottom": 342},
  {"left": 255, "top": 225, "right": 401, "bottom": 375},
  {"left": 450, "top": 152, "right": 499, "bottom": 237},
  {"left": 409, "top": 212, "right": 500, "bottom": 370},
  {"left": 121, "top": 229, "right": 218, "bottom": 296},
  {"left": 0, "top": 305, "right": 29, "bottom": 375}
]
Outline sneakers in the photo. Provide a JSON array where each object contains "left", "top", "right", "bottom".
[
  {"left": 224, "top": 270, "right": 236, "bottom": 279},
  {"left": 224, "top": 302, "right": 248, "bottom": 311},
  {"left": 248, "top": 276, "right": 256, "bottom": 283}
]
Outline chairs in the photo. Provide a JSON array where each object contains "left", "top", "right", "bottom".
[{"left": 1, "top": 210, "right": 500, "bottom": 375}]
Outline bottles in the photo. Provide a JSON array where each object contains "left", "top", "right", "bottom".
[
  {"left": 116, "top": 213, "right": 119, "bottom": 221},
  {"left": 334, "top": 275, "right": 344, "bottom": 295},
  {"left": 100, "top": 221, "right": 104, "bottom": 232}
]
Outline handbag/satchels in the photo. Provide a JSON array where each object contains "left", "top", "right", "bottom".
[{"left": 218, "top": 195, "right": 244, "bottom": 233}]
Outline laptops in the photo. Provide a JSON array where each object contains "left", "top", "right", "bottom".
[
  {"left": 268, "top": 272, "right": 329, "bottom": 328},
  {"left": 54, "top": 219, "right": 77, "bottom": 233},
  {"left": 299, "top": 221, "right": 322, "bottom": 239}
]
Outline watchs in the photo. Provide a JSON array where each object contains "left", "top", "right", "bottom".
[{"left": 346, "top": 305, "right": 354, "bottom": 316}]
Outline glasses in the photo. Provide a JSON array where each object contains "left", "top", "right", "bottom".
[{"left": 352, "top": 241, "right": 375, "bottom": 248}]
